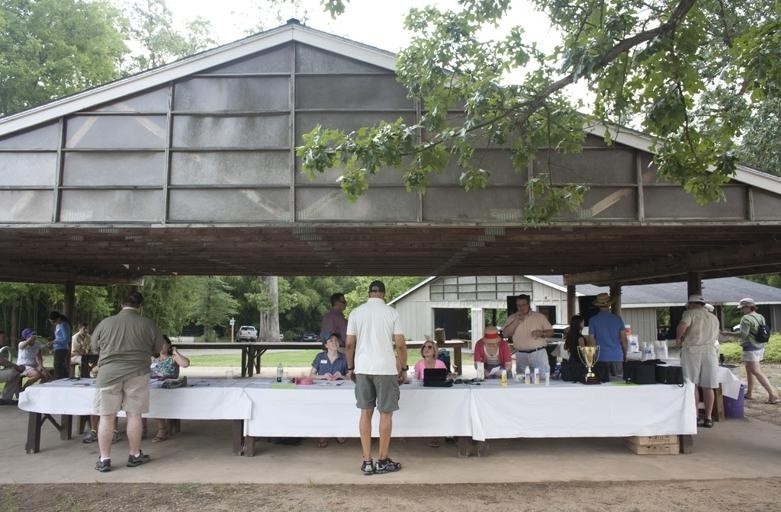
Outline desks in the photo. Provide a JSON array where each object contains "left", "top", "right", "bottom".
[
  {"left": 657, "top": 359, "right": 741, "bottom": 423},
  {"left": 244, "top": 378, "right": 473, "bottom": 457},
  {"left": 172, "top": 342, "right": 248, "bottom": 377},
  {"left": 18, "top": 378, "right": 244, "bottom": 456},
  {"left": 468, "top": 375, "right": 693, "bottom": 458},
  {"left": 248, "top": 339, "right": 465, "bottom": 377}
]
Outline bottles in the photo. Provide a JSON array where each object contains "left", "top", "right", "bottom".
[
  {"left": 534, "top": 367, "right": 539, "bottom": 384},
  {"left": 502, "top": 368, "right": 507, "bottom": 386},
  {"left": 544, "top": 369, "right": 550, "bottom": 384},
  {"left": 276, "top": 363, "right": 284, "bottom": 384},
  {"left": 646, "top": 343, "right": 651, "bottom": 359},
  {"left": 525, "top": 365, "right": 530, "bottom": 383}
]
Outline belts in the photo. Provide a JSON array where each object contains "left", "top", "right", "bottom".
[{"left": 518, "top": 346, "right": 546, "bottom": 353}]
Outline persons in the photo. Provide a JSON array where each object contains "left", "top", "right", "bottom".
[
  {"left": 71, "top": 323, "right": 92, "bottom": 365},
  {"left": 473, "top": 325, "right": 513, "bottom": 379},
  {"left": 673, "top": 293, "right": 720, "bottom": 428},
  {"left": 588, "top": 293, "right": 629, "bottom": 377},
  {"left": 90, "top": 291, "right": 163, "bottom": 473},
  {"left": 503, "top": 294, "right": 555, "bottom": 379},
  {"left": 308, "top": 331, "right": 348, "bottom": 449},
  {"left": 321, "top": 292, "right": 349, "bottom": 353},
  {"left": 149, "top": 331, "right": 191, "bottom": 443},
  {"left": 0, "top": 330, "right": 26, "bottom": 405},
  {"left": 81, "top": 366, "right": 122, "bottom": 445},
  {"left": 563, "top": 315, "right": 588, "bottom": 369},
  {"left": 48, "top": 311, "right": 73, "bottom": 379},
  {"left": 16, "top": 328, "right": 49, "bottom": 391},
  {"left": 719, "top": 297, "right": 781, "bottom": 405},
  {"left": 414, "top": 340, "right": 448, "bottom": 448},
  {"left": 344, "top": 281, "right": 409, "bottom": 475}
]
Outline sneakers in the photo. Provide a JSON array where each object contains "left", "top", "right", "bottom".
[
  {"left": 360, "top": 460, "right": 375, "bottom": 475},
  {"left": 94, "top": 455, "right": 111, "bottom": 472},
  {"left": 152, "top": 435, "right": 169, "bottom": 443},
  {"left": 375, "top": 458, "right": 401, "bottom": 474},
  {"left": 127, "top": 450, "right": 150, "bottom": 467},
  {"left": 84, "top": 429, "right": 98, "bottom": 444},
  {"left": 698, "top": 418, "right": 713, "bottom": 428},
  {"left": 111, "top": 430, "right": 122, "bottom": 444}
]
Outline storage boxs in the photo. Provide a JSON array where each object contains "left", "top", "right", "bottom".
[{"left": 624, "top": 435, "right": 679, "bottom": 455}]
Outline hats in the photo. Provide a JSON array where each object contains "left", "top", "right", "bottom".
[
  {"left": 738, "top": 298, "right": 754, "bottom": 309},
  {"left": 481, "top": 326, "right": 501, "bottom": 343},
  {"left": 688, "top": 294, "right": 706, "bottom": 303},
  {"left": 592, "top": 293, "right": 616, "bottom": 307},
  {"left": 22, "top": 328, "right": 35, "bottom": 337},
  {"left": 322, "top": 331, "right": 340, "bottom": 340},
  {"left": 368, "top": 280, "right": 385, "bottom": 292}
]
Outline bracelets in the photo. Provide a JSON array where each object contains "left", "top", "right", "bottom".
[{"left": 346, "top": 367, "right": 353, "bottom": 370}]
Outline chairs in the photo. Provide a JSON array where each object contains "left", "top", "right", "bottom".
[{"left": 78, "top": 355, "right": 118, "bottom": 435}]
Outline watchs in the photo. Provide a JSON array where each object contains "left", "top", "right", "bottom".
[
  {"left": 401, "top": 365, "right": 409, "bottom": 371},
  {"left": 541, "top": 330, "right": 546, "bottom": 338}
]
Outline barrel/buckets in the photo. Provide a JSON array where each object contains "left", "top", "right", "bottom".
[{"left": 724, "top": 384, "right": 745, "bottom": 419}]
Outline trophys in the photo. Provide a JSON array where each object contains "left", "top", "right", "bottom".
[{"left": 576, "top": 344, "right": 601, "bottom": 384}]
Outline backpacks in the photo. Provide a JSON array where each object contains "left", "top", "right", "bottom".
[{"left": 749, "top": 315, "right": 771, "bottom": 343}]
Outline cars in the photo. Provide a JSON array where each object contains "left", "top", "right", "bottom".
[
  {"left": 296, "top": 331, "right": 317, "bottom": 342},
  {"left": 237, "top": 325, "right": 258, "bottom": 341}
]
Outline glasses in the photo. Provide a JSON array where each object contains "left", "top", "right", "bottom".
[
  {"left": 339, "top": 300, "right": 346, "bottom": 304},
  {"left": 423, "top": 345, "right": 434, "bottom": 350}
]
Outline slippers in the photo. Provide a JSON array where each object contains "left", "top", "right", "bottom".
[
  {"left": 318, "top": 441, "right": 328, "bottom": 448},
  {"left": 765, "top": 399, "right": 781, "bottom": 405},
  {"left": 429, "top": 440, "right": 440, "bottom": 447},
  {"left": 335, "top": 438, "right": 346, "bottom": 444},
  {"left": 744, "top": 395, "right": 751, "bottom": 399}
]
